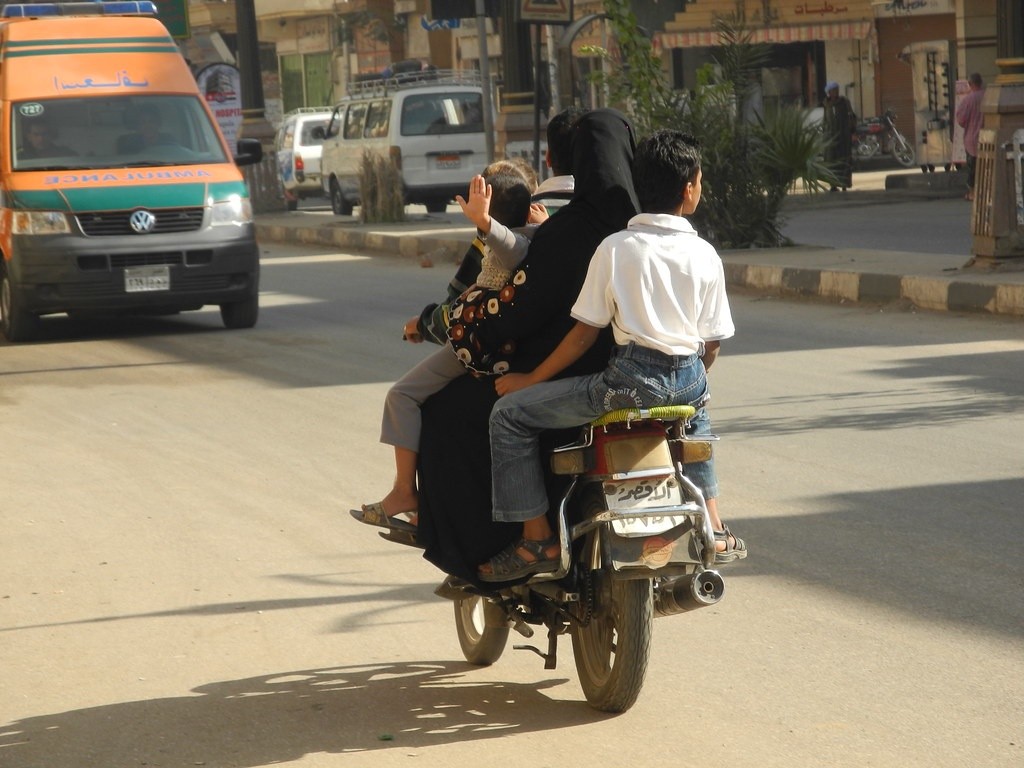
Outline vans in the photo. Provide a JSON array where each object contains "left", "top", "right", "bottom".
[
  {"left": 1, "top": 1, "right": 257, "bottom": 337},
  {"left": 309, "top": 75, "right": 495, "bottom": 223},
  {"left": 270, "top": 101, "right": 336, "bottom": 200}
]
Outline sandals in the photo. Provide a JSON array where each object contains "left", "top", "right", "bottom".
[
  {"left": 476, "top": 538, "right": 562, "bottom": 582},
  {"left": 703, "top": 523, "right": 746, "bottom": 563}
]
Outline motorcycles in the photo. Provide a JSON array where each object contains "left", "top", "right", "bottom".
[
  {"left": 403, "top": 311, "right": 722, "bottom": 714},
  {"left": 856, "top": 111, "right": 918, "bottom": 168}
]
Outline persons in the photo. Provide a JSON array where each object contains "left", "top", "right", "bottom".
[
  {"left": 956, "top": 72, "right": 986, "bottom": 202},
  {"left": 114, "top": 103, "right": 178, "bottom": 158},
  {"left": 347, "top": 104, "right": 750, "bottom": 592},
  {"left": 822, "top": 81, "right": 857, "bottom": 194},
  {"left": 16, "top": 116, "right": 81, "bottom": 161}
]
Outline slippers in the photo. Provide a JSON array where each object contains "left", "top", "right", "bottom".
[
  {"left": 350, "top": 503, "right": 418, "bottom": 535},
  {"left": 378, "top": 529, "right": 427, "bottom": 550}
]
